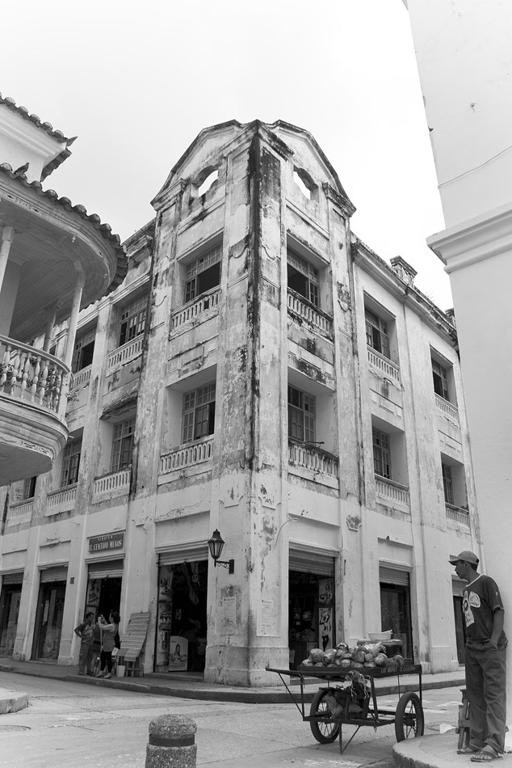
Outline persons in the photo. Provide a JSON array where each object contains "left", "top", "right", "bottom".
[
  {"left": 174, "top": 644, "right": 184, "bottom": 664},
  {"left": 102, "top": 630, "right": 120, "bottom": 655},
  {"left": 96, "top": 610, "right": 120, "bottom": 679},
  {"left": 74, "top": 612, "right": 96, "bottom": 677},
  {"left": 448, "top": 549, "right": 509, "bottom": 762}
]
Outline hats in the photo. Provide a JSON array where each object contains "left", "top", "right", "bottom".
[{"left": 448, "top": 551, "right": 479, "bottom": 565}]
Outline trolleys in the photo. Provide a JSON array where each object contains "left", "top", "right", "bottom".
[{"left": 262, "top": 662, "right": 426, "bottom": 759}]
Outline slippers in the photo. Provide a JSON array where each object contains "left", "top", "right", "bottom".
[
  {"left": 457, "top": 744, "right": 479, "bottom": 754},
  {"left": 471, "top": 750, "right": 503, "bottom": 762}
]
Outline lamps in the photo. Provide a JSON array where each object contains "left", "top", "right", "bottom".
[{"left": 207, "top": 527, "right": 234, "bottom": 575}]
